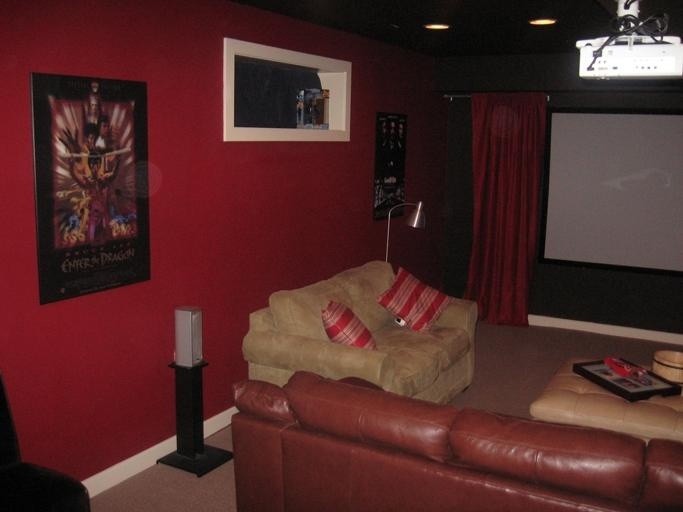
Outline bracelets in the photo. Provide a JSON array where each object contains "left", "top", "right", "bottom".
[{"left": 651, "top": 349, "right": 682, "bottom": 385}]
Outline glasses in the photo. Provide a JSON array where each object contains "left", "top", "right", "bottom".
[{"left": 385, "top": 199, "right": 427, "bottom": 262}]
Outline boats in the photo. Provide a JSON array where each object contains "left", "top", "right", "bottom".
[{"left": 174, "top": 305, "right": 204, "bottom": 368}]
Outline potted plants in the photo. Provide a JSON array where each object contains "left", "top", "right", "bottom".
[{"left": 571, "top": 354, "right": 682, "bottom": 402}]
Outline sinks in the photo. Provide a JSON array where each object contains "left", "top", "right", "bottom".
[
  {"left": 238, "top": 258, "right": 481, "bottom": 408},
  {"left": 228, "top": 367, "right": 683, "bottom": 511}
]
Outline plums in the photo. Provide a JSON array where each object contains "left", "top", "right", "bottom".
[{"left": 575, "top": 34, "right": 683, "bottom": 81}]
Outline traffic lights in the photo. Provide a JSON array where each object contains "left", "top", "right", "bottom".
[
  {"left": 322, "top": 298, "right": 378, "bottom": 354},
  {"left": 374, "top": 267, "right": 455, "bottom": 337}
]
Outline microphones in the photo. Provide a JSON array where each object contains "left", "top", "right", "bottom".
[{"left": 528, "top": 357, "right": 683, "bottom": 443}]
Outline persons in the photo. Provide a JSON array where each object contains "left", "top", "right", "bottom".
[
  {"left": 83, "top": 123, "right": 99, "bottom": 147},
  {"left": 99, "top": 114, "right": 112, "bottom": 147},
  {"left": 58, "top": 125, "right": 120, "bottom": 243}
]
